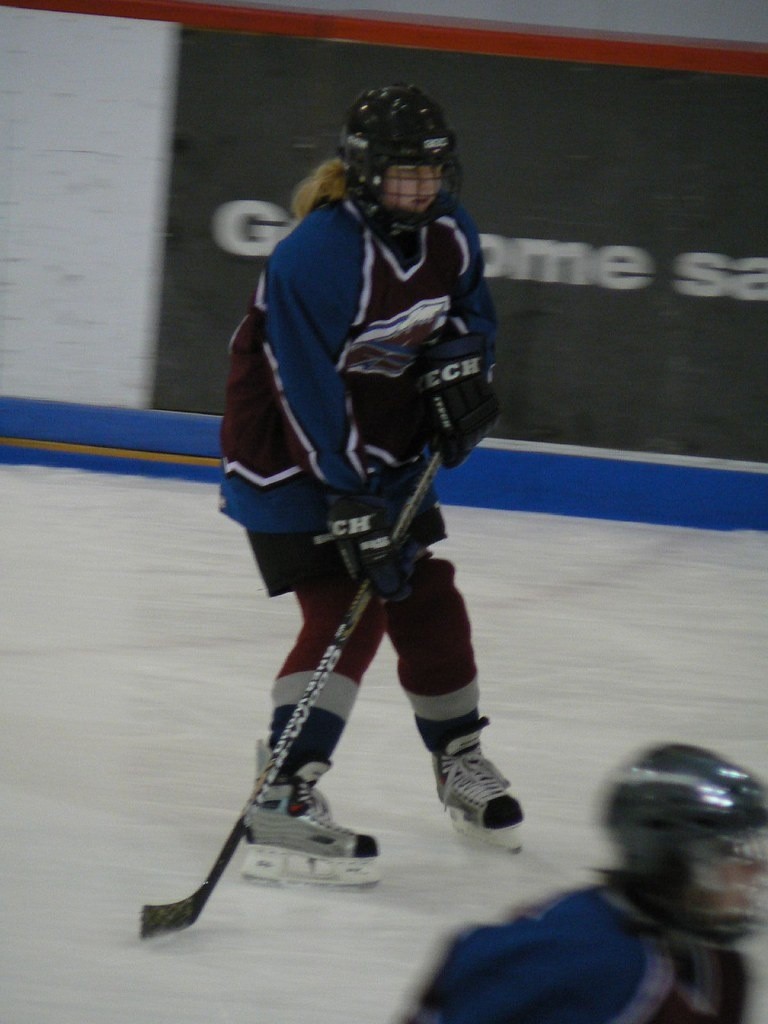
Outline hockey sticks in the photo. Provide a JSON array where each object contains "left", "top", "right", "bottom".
[{"left": 136, "top": 441, "right": 447, "bottom": 944}]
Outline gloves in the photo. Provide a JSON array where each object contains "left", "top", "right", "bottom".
[
  {"left": 324, "top": 496, "right": 425, "bottom": 601},
  {"left": 415, "top": 353, "right": 501, "bottom": 467}
]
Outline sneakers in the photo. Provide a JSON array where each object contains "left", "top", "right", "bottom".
[
  {"left": 240, "top": 739, "right": 382, "bottom": 890},
  {"left": 432, "top": 717, "right": 525, "bottom": 855}
]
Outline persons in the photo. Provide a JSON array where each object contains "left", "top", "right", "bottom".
[
  {"left": 218, "top": 81, "right": 524, "bottom": 859},
  {"left": 393, "top": 741, "right": 768, "bottom": 1024}
]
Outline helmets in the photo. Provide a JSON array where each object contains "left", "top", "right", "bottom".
[
  {"left": 604, "top": 741, "right": 768, "bottom": 942},
  {"left": 337, "top": 83, "right": 455, "bottom": 241}
]
